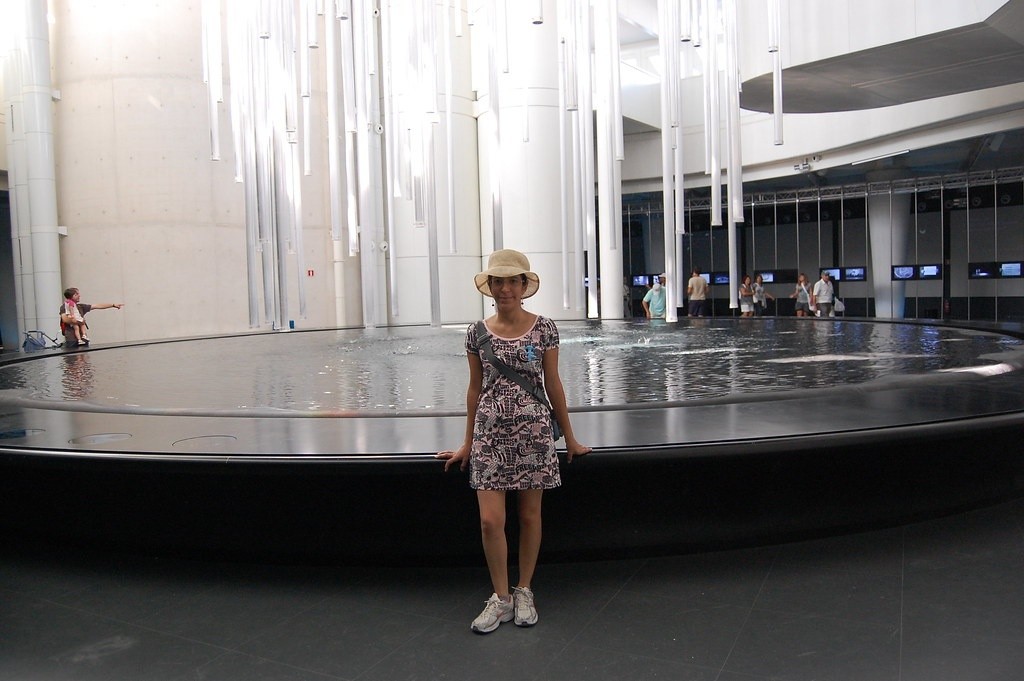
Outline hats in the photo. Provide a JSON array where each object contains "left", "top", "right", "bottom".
[
  {"left": 474, "top": 249, "right": 540, "bottom": 299},
  {"left": 821, "top": 271, "right": 830, "bottom": 275},
  {"left": 659, "top": 273, "right": 666, "bottom": 278},
  {"left": 653, "top": 283, "right": 661, "bottom": 295}
]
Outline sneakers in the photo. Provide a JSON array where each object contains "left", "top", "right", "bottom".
[
  {"left": 511, "top": 586, "right": 538, "bottom": 626},
  {"left": 471, "top": 592, "right": 514, "bottom": 633}
]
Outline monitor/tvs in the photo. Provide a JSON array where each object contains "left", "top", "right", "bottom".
[
  {"left": 891, "top": 261, "right": 1024, "bottom": 281},
  {"left": 631, "top": 271, "right": 729, "bottom": 287},
  {"left": 584, "top": 276, "right": 589, "bottom": 288},
  {"left": 754, "top": 267, "right": 867, "bottom": 284}
]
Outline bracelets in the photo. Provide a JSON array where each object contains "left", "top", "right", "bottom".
[{"left": 112, "top": 304, "right": 115, "bottom": 307}]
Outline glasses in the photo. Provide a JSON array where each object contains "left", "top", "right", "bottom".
[{"left": 489, "top": 278, "right": 526, "bottom": 287}]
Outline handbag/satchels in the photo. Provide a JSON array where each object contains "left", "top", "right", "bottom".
[
  {"left": 752, "top": 289, "right": 758, "bottom": 303},
  {"left": 834, "top": 296, "right": 845, "bottom": 312},
  {"left": 807, "top": 294, "right": 814, "bottom": 311},
  {"left": 550, "top": 409, "right": 564, "bottom": 442},
  {"left": 739, "top": 284, "right": 746, "bottom": 299}
]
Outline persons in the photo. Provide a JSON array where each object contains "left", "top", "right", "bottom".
[
  {"left": 59, "top": 288, "right": 124, "bottom": 345},
  {"left": 622, "top": 266, "right": 837, "bottom": 319},
  {"left": 435, "top": 250, "right": 593, "bottom": 634}
]
